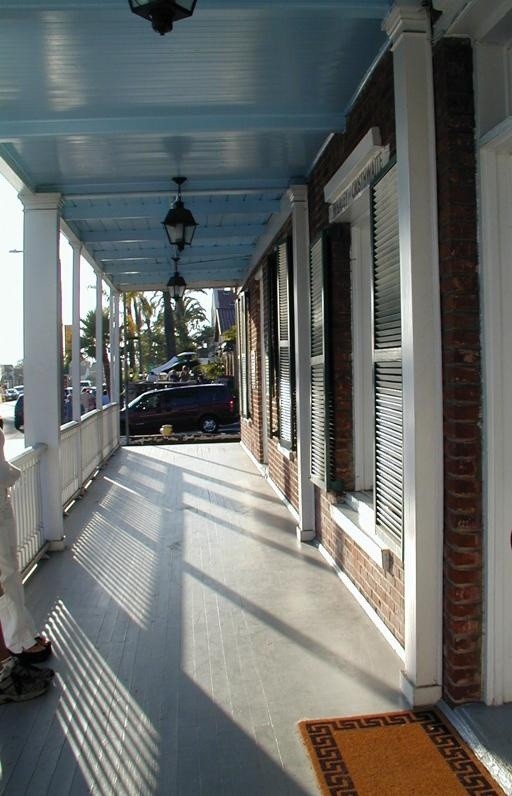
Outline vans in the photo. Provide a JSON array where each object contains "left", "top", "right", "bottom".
[
  {"left": 64, "top": 379, "right": 108, "bottom": 399},
  {"left": 120, "top": 381, "right": 241, "bottom": 437}
]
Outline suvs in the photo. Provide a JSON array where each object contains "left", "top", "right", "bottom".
[{"left": 14, "top": 394, "right": 24, "bottom": 430}]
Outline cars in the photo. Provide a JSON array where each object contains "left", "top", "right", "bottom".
[{"left": 4, "top": 386, "right": 24, "bottom": 402}]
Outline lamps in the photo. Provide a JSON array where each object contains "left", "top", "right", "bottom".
[
  {"left": 166, "top": 255, "right": 187, "bottom": 301},
  {"left": 127, "top": 0, "right": 197, "bottom": 37},
  {"left": 162, "top": 176, "right": 200, "bottom": 253}
]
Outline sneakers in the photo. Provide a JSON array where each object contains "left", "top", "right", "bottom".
[{"left": 0, "top": 637, "right": 55, "bottom": 704}]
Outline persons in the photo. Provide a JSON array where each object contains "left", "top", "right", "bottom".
[
  {"left": 146, "top": 371, "right": 157, "bottom": 383},
  {"left": 167, "top": 365, "right": 189, "bottom": 388},
  {"left": 1, "top": 426, "right": 56, "bottom": 703}
]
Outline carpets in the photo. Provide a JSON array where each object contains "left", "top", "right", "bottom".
[{"left": 293, "top": 706, "right": 507, "bottom": 796}]
